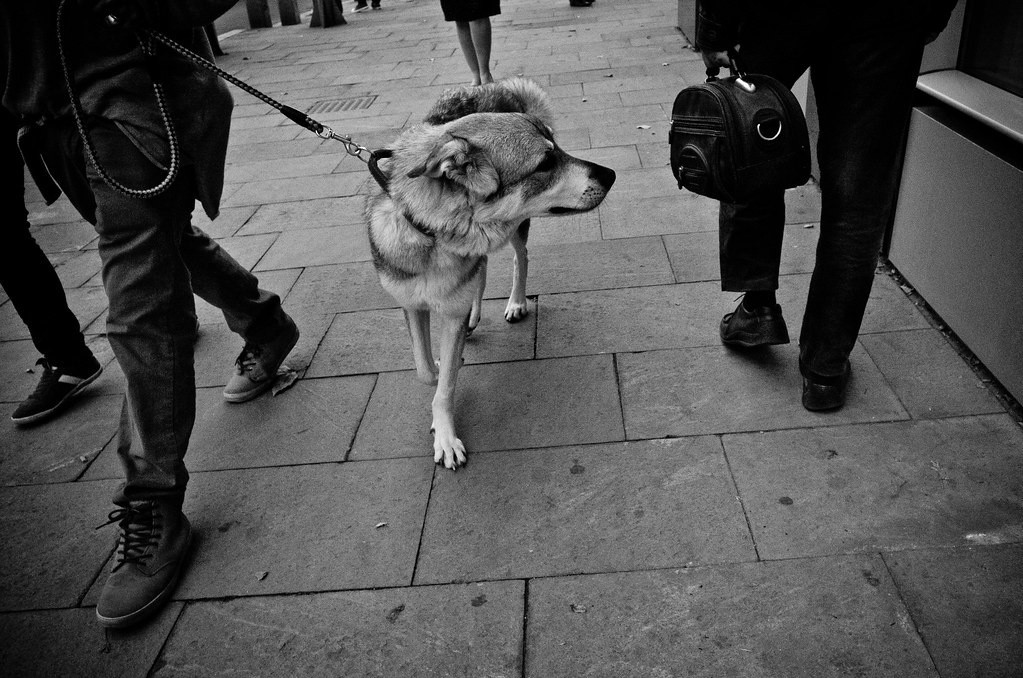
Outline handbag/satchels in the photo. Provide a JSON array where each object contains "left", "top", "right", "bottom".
[{"left": 670, "top": 47, "right": 812, "bottom": 209}]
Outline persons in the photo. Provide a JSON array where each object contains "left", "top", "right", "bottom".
[
  {"left": 695, "top": 0, "right": 959, "bottom": 415},
  {"left": 0, "top": 1, "right": 299, "bottom": 629},
  {"left": 0, "top": 111, "right": 101, "bottom": 428},
  {"left": 440, "top": 0, "right": 502, "bottom": 86},
  {"left": 352, "top": 0, "right": 384, "bottom": 13}
]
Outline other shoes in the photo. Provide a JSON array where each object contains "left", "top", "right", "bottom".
[
  {"left": 802, "top": 359, "right": 851, "bottom": 410},
  {"left": 373, "top": 5, "right": 381, "bottom": 10},
  {"left": 350, "top": 3, "right": 368, "bottom": 13},
  {"left": 719, "top": 293, "right": 790, "bottom": 349}
]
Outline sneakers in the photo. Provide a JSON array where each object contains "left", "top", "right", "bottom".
[
  {"left": 95, "top": 503, "right": 193, "bottom": 628},
  {"left": 223, "top": 313, "right": 300, "bottom": 403},
  {"left": 10, "top": 347, "right": 102, "bottom": 426}
]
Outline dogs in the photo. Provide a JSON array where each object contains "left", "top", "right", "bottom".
[{"left": 360, "top": 74, "right": 617, "bottom": 470}]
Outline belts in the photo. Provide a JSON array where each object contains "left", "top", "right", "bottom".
[{"left": 15, "top": 113, "right": 63, "bottom": 206}]
